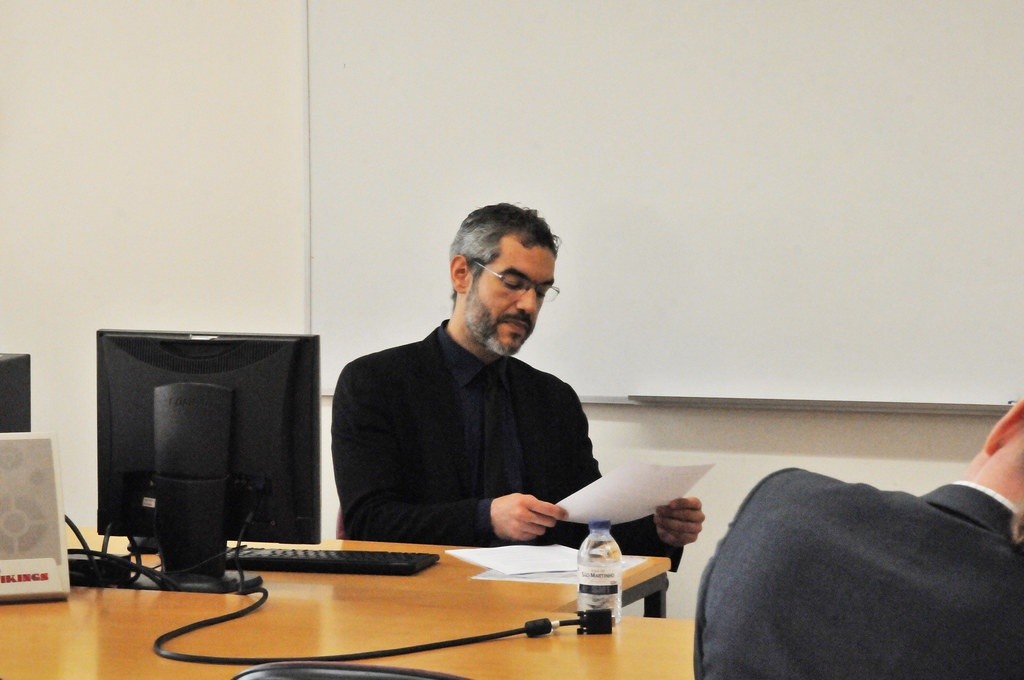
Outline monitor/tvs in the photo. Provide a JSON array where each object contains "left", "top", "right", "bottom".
[{"left": 96, "top": 330, "right": 322, "bottom": 592}]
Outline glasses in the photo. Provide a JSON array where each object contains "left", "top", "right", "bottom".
[{"left": 473, "top": 261, "right": 560, "bottom": 302}]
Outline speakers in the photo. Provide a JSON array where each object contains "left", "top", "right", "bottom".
[{"left": 0, "top": 432, "right": 71, "bottom": 604}]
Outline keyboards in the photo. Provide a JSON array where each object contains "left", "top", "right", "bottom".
[{"left": 224, "top": 546, "right": 440, "bottom": 576}]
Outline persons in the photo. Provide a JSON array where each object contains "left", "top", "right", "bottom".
[
  {"left": 333, "top": 203, "right": 707, "bottom": 573},
  {"left": 689, "top": 395, "right": 1024, "bottom": 680}
]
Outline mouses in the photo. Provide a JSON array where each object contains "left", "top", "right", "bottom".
[{"left": 126, "top": 535, "right": 159, "bottom": 554}]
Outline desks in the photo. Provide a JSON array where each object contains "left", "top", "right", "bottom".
[{"left": 0, "top": 530, "right": 696, "bottom": 680}]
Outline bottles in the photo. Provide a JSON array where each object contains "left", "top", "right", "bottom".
[{"left": 576, "top": 519, "right": 624, "bottom": 624}]
